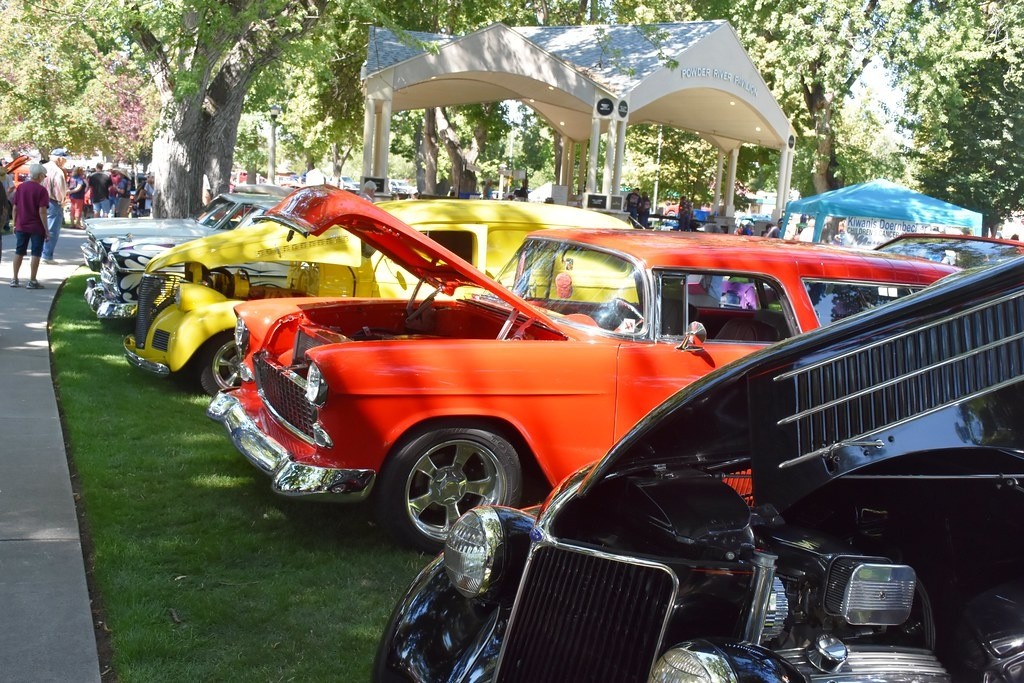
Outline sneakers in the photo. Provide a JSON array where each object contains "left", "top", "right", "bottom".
[
  {"left": 9, "top": 279, "right": 19, "bottom": 287},
  {"left": 26, "top": 280, "right": 44, "bottom": 288}
]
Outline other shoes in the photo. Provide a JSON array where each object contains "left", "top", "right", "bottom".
[
  {"left": 41, "top": 258, "right": 59, "bottom": 265},
  {"left": 76, "top": 225, "right": 83, "bottom": 228},
  {"left": 69, "top": 225, "right": 74, "bottom": 228}
]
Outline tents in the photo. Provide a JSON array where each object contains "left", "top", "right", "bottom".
[{"left": 779, "top": 179, "right": 982, "bottom": 250}]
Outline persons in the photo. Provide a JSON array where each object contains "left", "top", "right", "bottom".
[
  {"left": 407, "top": 189, "right": 418, "bottom": 199},
  {"left": 39, "top": 149, "right": 69, "bottom": 265},
  {"left": 66, "top": 163, "right": 155, "bottom": 230},
  {"left": 679, "top": 196, "right": 692, "bottom": 231},
  {"left": 736, "top": 217, "right": 784, "bottom": 238},
  {"left": 0, "top": 158, "right": 16, "bottom": 264},
  {"left": 1001, "top": 234, "right": 1022, "bottom": 256},
  {"left": 10, "top": 164, "right": 50, "bottom": 288},
  {"left": 792, "top": 217, "right": 857, "bottom": 247},
  {"left": 359, "top": 181, "right": 377, "bottom": 203},
  {"left": 516, "top": 179, "right": 528, "bottom": 198},
  {"left": 202, "top": 170, "right": 211, "bottom": 206},
  {"left": 624, "top": 188, "right": 651, "bottom": 229},
  {"left": 483, "top": 179, "right": 493, "bottom": 200}
]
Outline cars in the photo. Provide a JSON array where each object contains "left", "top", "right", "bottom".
[
  {"left": 371, "top": 256, "right": 1024, "bottom": 683},
  {"left": 119, "top": 199, "right": 645, "bottom": 400},
  {"left": 79, "top": 185, "right": 292, "bottom": 320},
  {"left": 876, "top": 231, "right": 1024, "bottom": 272},
  {"left": 205, "top": 184, "right": 964, "bottom": 546}
]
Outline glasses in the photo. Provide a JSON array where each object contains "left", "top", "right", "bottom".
[
  {"left": 41, "top": 172, "right": 47, "bottom": 178},
  {"left": 3, "top": 161, "right": 8, "bottom": 164}
]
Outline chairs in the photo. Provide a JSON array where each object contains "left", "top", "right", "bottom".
[
  {"left": 662, "top": 299, "right": 697, "bottom": 335},
  {"left": 753, "top": 309, "right": 795, "bottom": 340},
  {"left": 715, "top": 318, "right": 780, "bottom": 342}
]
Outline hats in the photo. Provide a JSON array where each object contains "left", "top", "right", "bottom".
[
  {"left": 543, "top": 197, "right": 554, "bottom": 203},
  {"left": 87, "top": 168, "right": 96, "bottom": 172},
  {"left": 778, "top": 218, "right": 784, "bottom": 223},
  {"left": 50, "top": 148, "right": 69, "bottom": 156},
  {"left": 113, "top": 167, "right": 131, "bottom": 180},
  {"left": 745, "top": 221, "right": 756, "bottom": 226}
]
